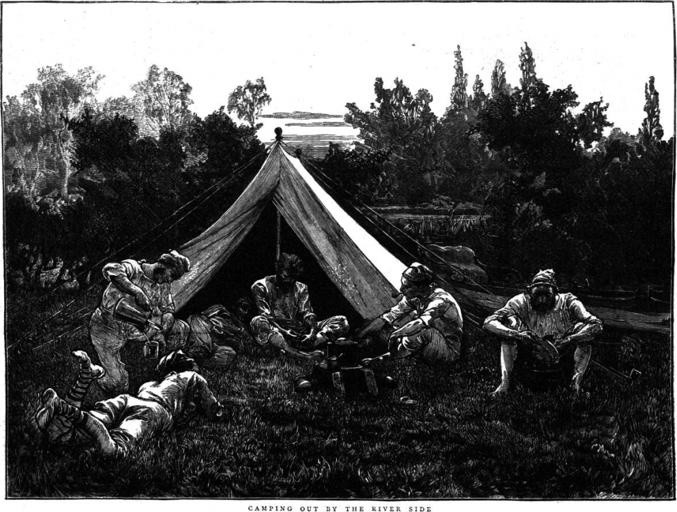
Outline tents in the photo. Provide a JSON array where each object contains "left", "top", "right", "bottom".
[{"left": 171, "top": 140, "right": 417, "bottom": 344}]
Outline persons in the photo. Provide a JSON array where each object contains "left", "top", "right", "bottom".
[
  {"left": 89, "top": 249, "right": 190, "bottom": 393},
  {"left": 357, "top": 262, "right": 463, "bottom": 368},
  {"left": 35, "top": 350, "right": 227, "bottom": 468},
  {"left": 164, "top": 294, "right": 252, "bottom": 371},
  {"left": 251, "top": 253, "right": 350, "bottom": 359},
  {"left": 482, "top": 269, "right": 604, "bottom": 396}
]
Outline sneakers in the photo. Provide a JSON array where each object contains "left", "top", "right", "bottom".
[
  {"left": 71, "top": 349, "right": 105, "bottom": 379},
  {"left": 37, "top": 387, "right": 67, "bottom": 431}
]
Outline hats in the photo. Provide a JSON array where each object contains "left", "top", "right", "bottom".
[
  {"left": 160, "top": 251, "right": 191, "bottom": 278},
  {"left": 404, "top": 262, "right": 433, "bottom": 284},
  {"left": 156, "top": 350, "right": 195, "bottom": 373},
  {"left": 526, "top": 267, "right": 560, "bottom": 291}
]
